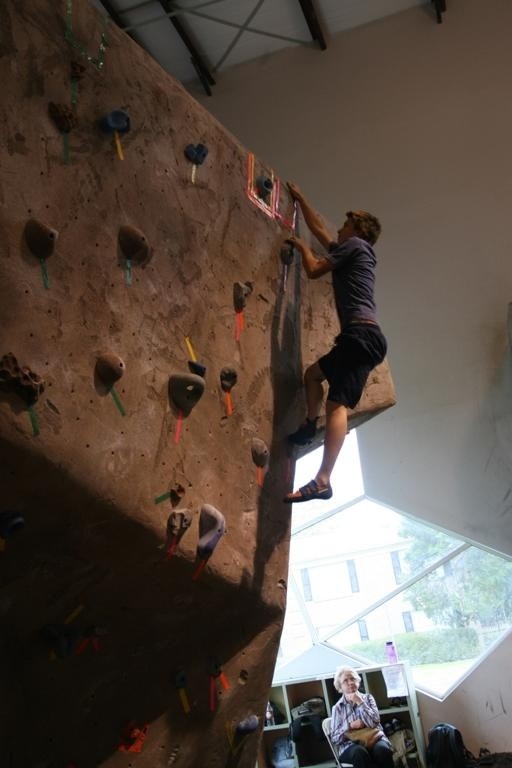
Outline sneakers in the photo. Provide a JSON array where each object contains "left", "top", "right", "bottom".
[{"left": 384, "top": 718, "right": 401, "bottom": 736}]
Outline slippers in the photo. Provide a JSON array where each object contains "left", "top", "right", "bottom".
[{"left": 390, "top": 697, "right": 406, "bottom": 706}]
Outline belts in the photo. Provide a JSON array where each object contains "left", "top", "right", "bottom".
[{"left": 350, "top": 318, "right": 379, "bottom": 326}]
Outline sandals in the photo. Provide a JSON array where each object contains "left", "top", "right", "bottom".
[
  {"left": 283, "top": 477, "right": 332, "bottom": 502},
  {"left": 288, "top": 417, "right": 320, "bottom": 446}
]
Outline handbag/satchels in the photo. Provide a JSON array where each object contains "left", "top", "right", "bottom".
[
  {"left": 389, "top": 729, "right": 413, "bottom": 761},
  {"left": 344, "top": 727, "right": 384, "bottom": 748},
  {"left": 467, "top": 752, "right": 512, "bottom": 768}
]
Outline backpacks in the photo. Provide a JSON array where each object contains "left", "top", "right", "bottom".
[
  {"left": 426, "top": 722, "right": 464, "bottom": 767},
  {"left": 266, "top": 703, "right": 284, "bottom": 726},
  {"left": 272, "top": 698, "right": 333, "bottom": 767}
]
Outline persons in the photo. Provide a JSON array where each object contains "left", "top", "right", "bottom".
[
  {"left": 331, "top": 666, "right": 395, "bottom": 767},
  {"left": 282, "top": 175, "right": 388, "bottom": 504}
]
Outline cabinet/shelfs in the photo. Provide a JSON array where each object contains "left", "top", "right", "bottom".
[{"left": 255, "top": 660, "right": 427, "bottom": 768}]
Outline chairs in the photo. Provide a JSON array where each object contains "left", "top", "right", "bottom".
[
  {"left": 322, "top": 718, "right": 353, "bottom": 768},
  {"left": 426, "top": 722, "right": 494, "bottom": 768}
]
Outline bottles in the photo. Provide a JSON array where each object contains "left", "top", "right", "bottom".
[
  {"left": 265, "top": 700, "right": 276, "bottom": 727},
  {"left": 385, "top": 641, "right": 399, "bottom": 666}
]
